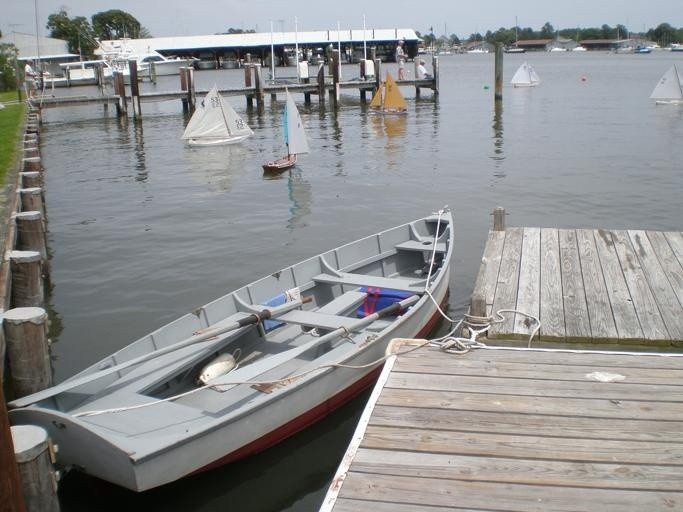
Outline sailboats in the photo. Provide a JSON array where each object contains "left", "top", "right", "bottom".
[
  {"left": 180, "top": 81, "right": 255, "bottom": 147},
  {"left": 509, "top": 59, "right": 541, "bottom": 88},
  {"left": 366, "top": 71, "right": 409, "bottom": 115},
  {"left": 261, "top": 87, "right": 309, "bottom": 174},
  {"left": 648, "top": 64, "right": 682, "bottom": 105}
]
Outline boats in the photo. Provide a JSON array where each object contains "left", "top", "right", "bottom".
[
  {"left": 2, "top": 203, "right": 454, "bottom": 496},
  {"left": 37, "top": 49, "right": 200, "bottom": 88}
]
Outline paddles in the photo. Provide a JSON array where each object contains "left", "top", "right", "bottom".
[
  {"left": 205, "top": 294, "right": 423, "bottom": 393},
  {"left": 8, "top": 298, "right": 313, "bottom": 408}
]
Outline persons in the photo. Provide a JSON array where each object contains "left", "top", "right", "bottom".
[
  {"left": 416, "top": 58, "right": 435, "bottom": 80},
  {"left": 23, "top": 58, "right": 38, "bottom": 100},
  {"left": 395, "top": 39, "right": 408, "bottom": 81}
]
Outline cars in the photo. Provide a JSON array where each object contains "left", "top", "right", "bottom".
[
  {"left": 466, "top": 43, "right": 495, "bottom": 53},
  {"left": 550, "top": 47, "right": 565, "bottom": 52},
  {"left": 572, "top": 47, "right": 586, "bottom": 51}
]
